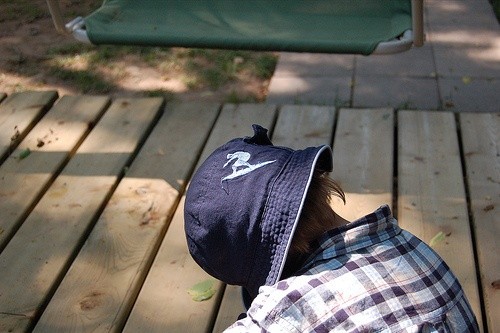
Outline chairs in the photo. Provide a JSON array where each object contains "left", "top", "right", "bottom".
[{"left": 45, "top": 0, "right": 430, "bottom": 58}]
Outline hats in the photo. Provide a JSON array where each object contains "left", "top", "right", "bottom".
[{"left": 184, "top": 123, "right": 334, "bottom": 307}]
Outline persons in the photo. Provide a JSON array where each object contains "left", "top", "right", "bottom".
[{"left": 182, "top": 124, "right": 480, "bottom": 333}]
